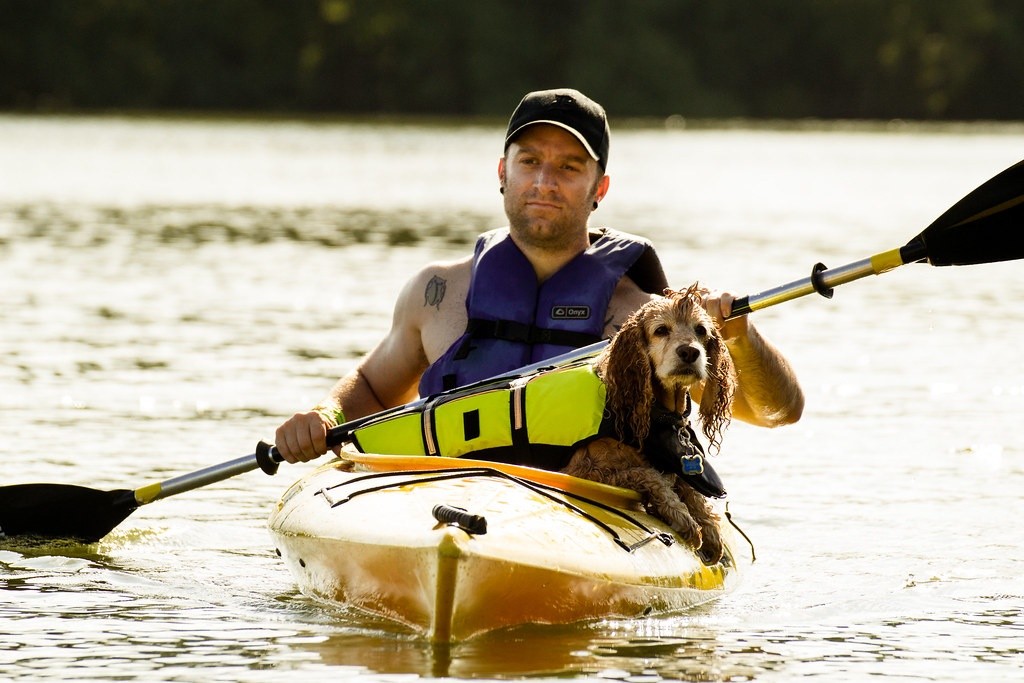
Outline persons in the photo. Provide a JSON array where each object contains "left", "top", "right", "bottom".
[{"left": 274, "top": 87, "right": 804, "bottom": 471}]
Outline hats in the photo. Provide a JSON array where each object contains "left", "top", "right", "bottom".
[{"left": 505, "top": 88, "right": 609, "bottom": 172}]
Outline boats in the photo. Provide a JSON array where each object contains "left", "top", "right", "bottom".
[{"left": 269, "top": 440, "right": 730, "bottom": 645}]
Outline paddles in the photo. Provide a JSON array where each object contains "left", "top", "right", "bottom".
[{"left": 0, "top": 160, "right": 1024, "bottom": 540}]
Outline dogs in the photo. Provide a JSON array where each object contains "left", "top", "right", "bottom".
[{"left": 559, "top": 284, "right": 736, "bottom": 571}]
